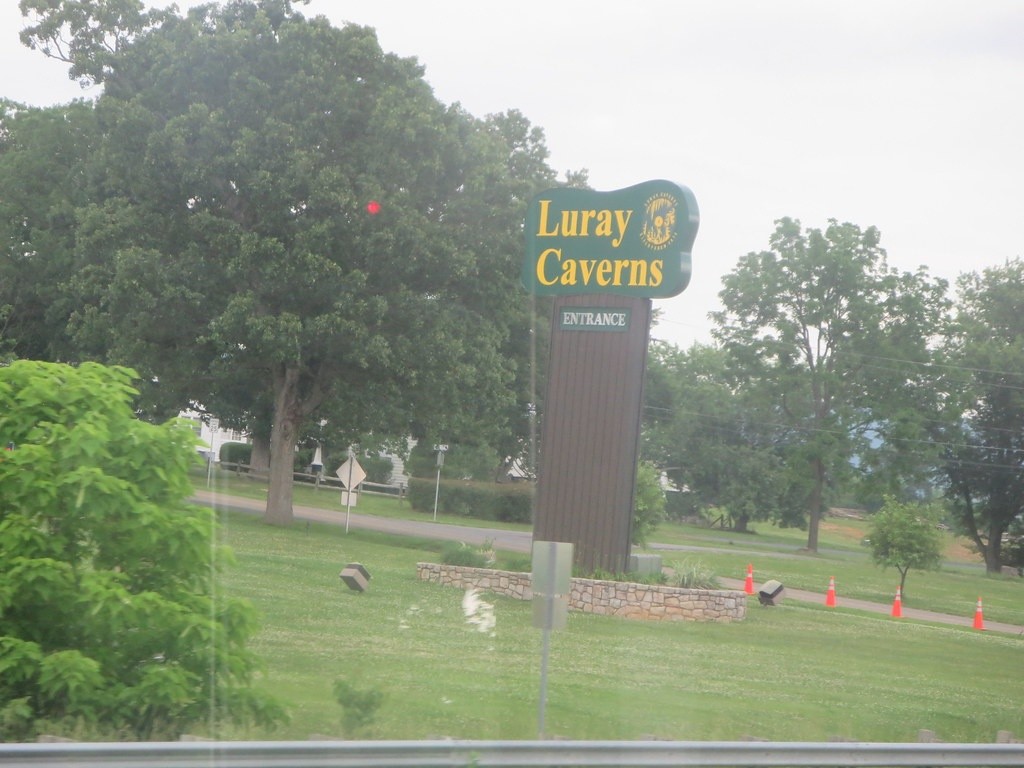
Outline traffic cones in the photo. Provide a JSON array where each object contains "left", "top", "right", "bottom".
[
  {"left": 891, "top": 585, "right": 903, "bottom": 618},
  {"left": 969, "top": 595, "right": 991, "bottom": 632},
  {"left": 744, "top": 559, "right": 757, "bottom": 595},
  {"left": 825, "top": 575, "right": 836, "bottom": 606}
]
